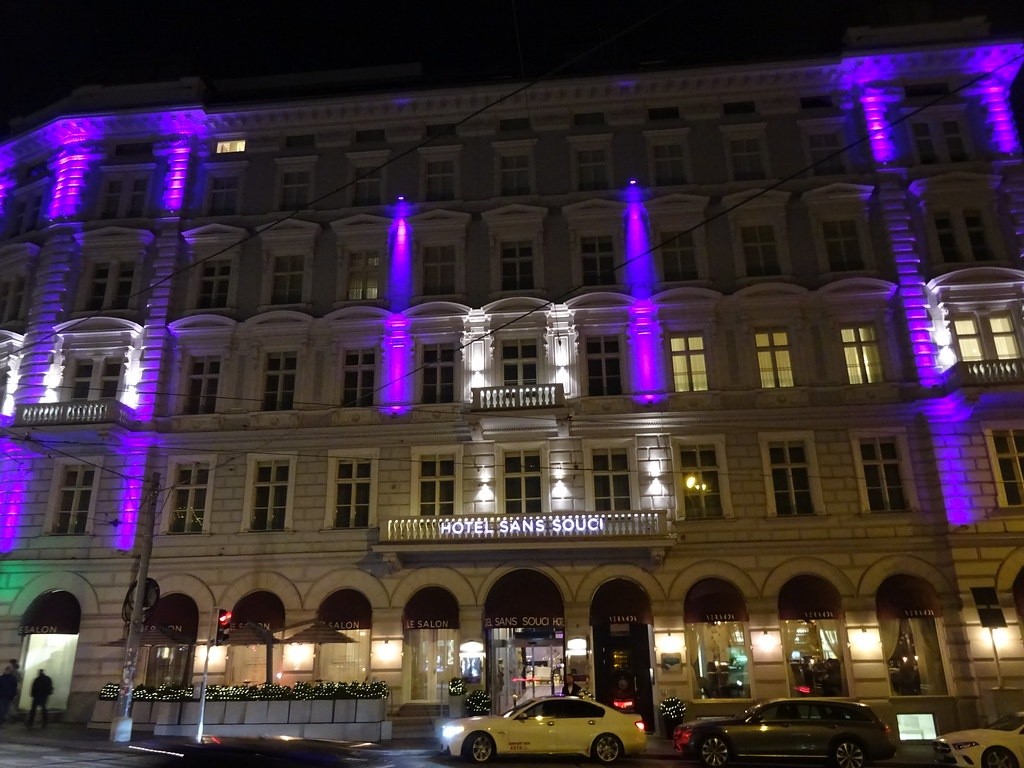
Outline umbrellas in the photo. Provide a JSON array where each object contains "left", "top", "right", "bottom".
[
  {"left": 284, "top": 623, "right": 360, "bottom": 644},
  {"left": 99, "top": 624, "right": 195, "bottom": 648},
  {"left": 204, "top": 623, "right": 285, "bottom": 646}
]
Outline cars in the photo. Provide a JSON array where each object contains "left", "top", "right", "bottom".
[
  {"left": 935, "top": 708, "right": 1024, "bottom": 768},
  {"left": 672, "top": 697, "right": 895, "bottom": 768},
  {"left": 438, "top": 693, "right": 647, "bottom": 764}
]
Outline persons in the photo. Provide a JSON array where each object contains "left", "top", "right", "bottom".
[
  {"left": 693, "top": 653, "right": 719, "bottom": 698},
  {"left": 0, "top": 666, "right": 18, "bottom": 721},
  {"left": 560, "top": 674, "right": 585, "bottom": 695},
  {"left": 24, "top": 669, "right": 54, "bottom": 729}
]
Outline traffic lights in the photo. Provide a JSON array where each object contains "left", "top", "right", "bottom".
[{"left": 213, "top": 607, "right": 232, "bottom": 645}]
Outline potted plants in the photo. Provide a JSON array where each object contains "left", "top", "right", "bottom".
[
  {"left": 448, "top": 677, "right": 468, "bottom": 718},
  {"left": 266, "top": 684, "right": 291, "bottom": 724},
  {"left": 204, "top": 683, "right": 226, "bottom": 724},
  {"left": 150, "top": 682, "right": 166, "bottom": 723},
  {"left": 156, "top": 684, "right": 182, "bottom": 725},
  {"left": 224, "top": 686, "right": 247, "bottom": 724},
  {"left": 333, "top": 682, "right": 356, "bottom": 723},
  {"left": 130, "top": 684, "right": 154, "bottom": 723},
  {"left": 355, "top": 686, "right": 384, "bottom": 722},
  {"left": 657, "top": 696, "right": 688, "bottom": 740},
  {"left": 310, "top": 683, "right": 334, "bottom": 723},
  {"left": 288, "top": 681, "right": 312, "bottom": 723},
  {"left": 244, "top": 685, "right": 269, "bottom": 724},
  {"left": 91, "top": 682, "right": 120, "bottom": 722},
  {"left": 379, "top": 680, "right": 390, "bottom": 721},
  {"left": 179, "top": 682, "right": 203, "bottom": 725}
]
